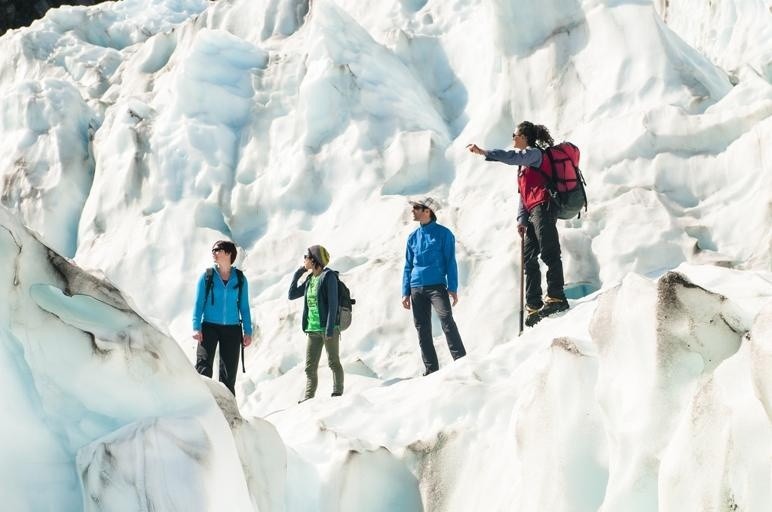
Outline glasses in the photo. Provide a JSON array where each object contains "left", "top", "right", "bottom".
[
  {"left": 303, "top": 254, "right": 310, "bottom": 259},
  {"left": 413, "top": 205, "right": 424, "bottom": 210},
  {"left": 211, "top": 248, "right": 220, "bottom": 253}
]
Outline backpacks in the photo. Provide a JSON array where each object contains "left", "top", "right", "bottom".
[
  {"left": 535, "top": 140, "right": 588, "bottom": 219},
  {"left": 335, "top": 271, "right": 355, "bottom": 331}
]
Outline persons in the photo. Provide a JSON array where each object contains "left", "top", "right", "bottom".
[
  {"left": 465, "top": 121, "right": 569, "bottom": 327},
  {"left": 401, "top": 198, "right": 466, "bottom": 376},
  {"left": 192, "top": 240, "right": 252, "bottom": 396},
  {"left": 288, "top": 245, "right": 344, "bottom": 403}
]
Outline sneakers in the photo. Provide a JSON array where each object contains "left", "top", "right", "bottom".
[
  {"left": 538, "top": 297, "right": 569, "bottom": 316},
  {"left": 524, "top": 304, "right": 545, "bottom": 325}
]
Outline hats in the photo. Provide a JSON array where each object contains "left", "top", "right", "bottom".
[
  {"left": 409, "top": 197, "right": 441, "bottom": 219},
  {"left": 308, "top": 244, "right": 330, "bottom": 267}
]
